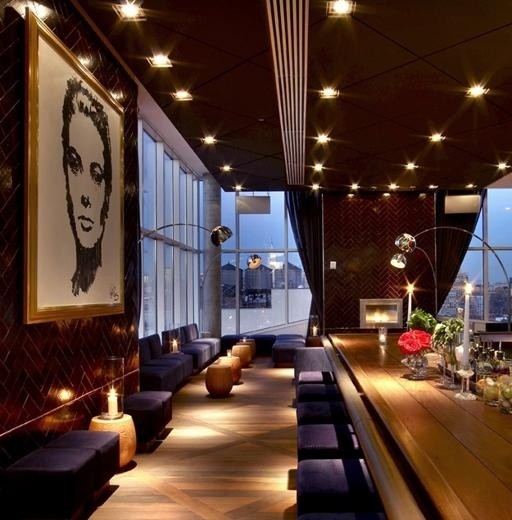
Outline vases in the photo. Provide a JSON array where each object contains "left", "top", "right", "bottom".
[{"left": 408, "top": 353, "right": 428, "bottom": 382}]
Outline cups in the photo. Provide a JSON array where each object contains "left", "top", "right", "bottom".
[
  {"left": 379, "top": 327, "right": 386, "bottom": 344},
  {"left": 227, "top": 350, "right": 231, "bottom": 359},
  {"left": 169, "top": 322, "right": 180, "bottom": 353},
  {"left": 102, "top": 355, "right": 124, "bottom": 420},
  {"left": 242, "top": 337, "right": 246, "bottom": 345}
]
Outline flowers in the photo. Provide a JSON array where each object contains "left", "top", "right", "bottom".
[
  {"left": 406, "top": 307, "right": 438, "bottom": 337},
  {"left": 397, "top": 330, "right": 435, "bottom": 354}
]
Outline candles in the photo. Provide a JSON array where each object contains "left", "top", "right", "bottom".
[
  {"left": 459, "top": 282, "right": 473, "bottom": 368},
  {"left": 404, "top": 282, "right": 415, "bottom": 323}
]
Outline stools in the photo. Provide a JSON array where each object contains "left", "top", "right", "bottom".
[
  {"left": 243, "top": 333, "right": 329, "bottom": 370},
  {"left": 6, "top": 430, "right": 120, "bottom": 512},
  {"left": 123, "top": 391, "right": 173, "bottom": 445},
  {"left": 294, "top": 372, "right": 374, "bottom": 513}
]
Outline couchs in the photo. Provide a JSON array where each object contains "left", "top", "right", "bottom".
[{"left": 138, "top": 324, "right": 242, "bottom": 393}]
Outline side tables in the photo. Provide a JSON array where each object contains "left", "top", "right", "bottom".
[{"left": 89, "top": 414, "right": 137, "bottom": 470}]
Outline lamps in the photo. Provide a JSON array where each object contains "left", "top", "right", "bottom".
[
  {"left": 140, "top": 221, "right": 235, "bottom": 248},
  {"left": 199, "top": 247, "right": 263, "bottom": 289},
  {"left": 393, "top": 224, "right": 511, "bottom": 289},
  {"left": 388, "top": 245, "right": 440, "bottom": 317}
]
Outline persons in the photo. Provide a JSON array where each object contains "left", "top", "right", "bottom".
[{"left": 60, "top": 76, "right": 112, "bottom": 296}]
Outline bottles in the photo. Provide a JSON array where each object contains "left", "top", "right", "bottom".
[{"left": 474, "top": 343, "right": 511, "bottom": 414}]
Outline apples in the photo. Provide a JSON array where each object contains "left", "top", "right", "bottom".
[{"left": 476, "top": 375, "right": 512, "bottom": 409}]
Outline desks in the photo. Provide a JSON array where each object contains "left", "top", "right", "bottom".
[{"left": 331, "top": 330, "right": 511, "bottom": 518}]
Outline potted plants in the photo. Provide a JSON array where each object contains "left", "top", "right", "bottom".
[{"left": 433, "top": 318, "right": 462, "bottom": 390}]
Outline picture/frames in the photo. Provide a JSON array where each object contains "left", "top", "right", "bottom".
[{"left": 24, "top": 4, "right": 128, "bottom": 327}]
